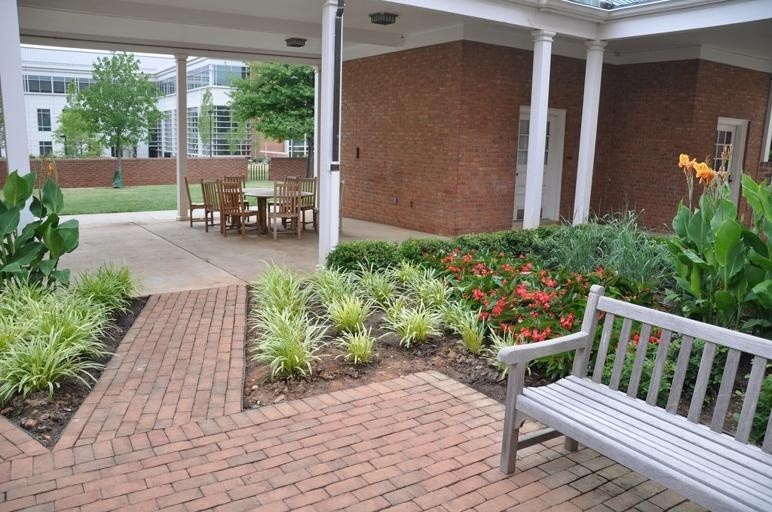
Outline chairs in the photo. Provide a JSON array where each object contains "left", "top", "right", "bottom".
[{"left": 184, "top": 174, "right": 345, "bottom": 241}]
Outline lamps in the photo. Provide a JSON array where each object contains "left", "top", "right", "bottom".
[
  {"left": 284, "top": 37, "right": 306, "bottom": 47},
  {"left": 368, "top": 11, "right": 398, "bottom": 25}
]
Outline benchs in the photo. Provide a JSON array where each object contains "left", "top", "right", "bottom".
[{"left": 496, "top": 283, "right": 772, "bottom": 512}]
